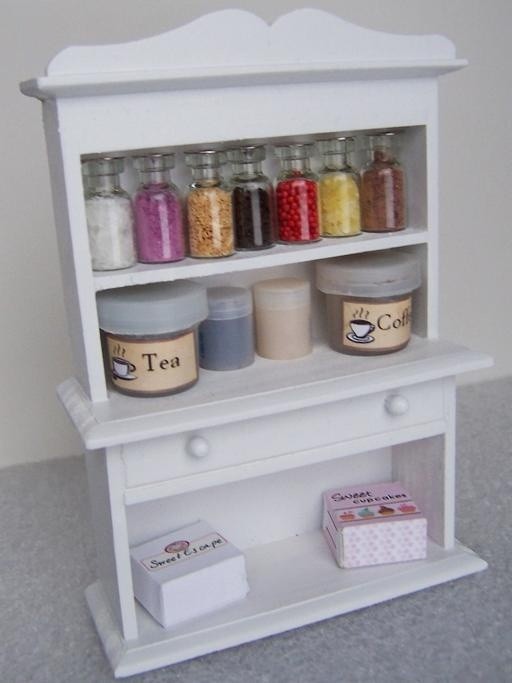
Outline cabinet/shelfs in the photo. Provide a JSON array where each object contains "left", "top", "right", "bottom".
[{"left": 19, "top": 8, "right": 489, "bottom": 680}]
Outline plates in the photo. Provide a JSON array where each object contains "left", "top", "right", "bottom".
[
  {"left": 346, "top": 332, "right": 375, "bottom": 344},
  {"left": 110, "top": 370, "right": 137, "bottom": 380}
]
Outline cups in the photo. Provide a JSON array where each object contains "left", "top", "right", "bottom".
[
  {"left": 349, "top": 319, "right": 375, "bottom": 337},
  {"left": 112, "top": 357, "right": 136, "bottom": 376}
]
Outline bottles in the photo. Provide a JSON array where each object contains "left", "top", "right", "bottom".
[
  {"left": 199, "top": 276, "right": 311, "bottom": 372},
  {"left": 321, "top": 136, "right": 366, "bottom": 238},
  {"left": 135, "top": 152, "right": 188, "bottom": 264},
  {"left": 235, "top": 139, "right": 274, "bottom": 252},
  {"left": 186, "top": 144, "right": 234, "bottom": 263},
  {"left": 79, "top": 154, "right": 135, "bottom": 272},
  {"left": 369, "top": 129, "right": 408, "bottom": 233},
  {"left": 277, "top": 133, "right": 322, "bottom": 245}
]
